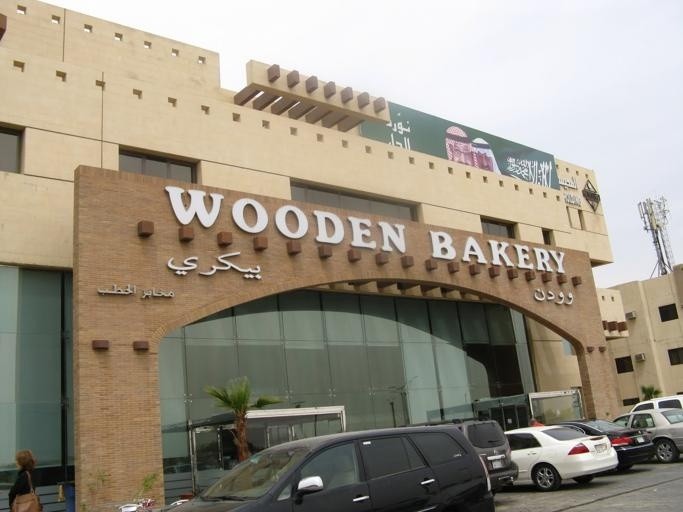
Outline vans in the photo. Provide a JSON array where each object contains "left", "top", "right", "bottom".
[{"left": 165, "top": 424, "right": 494, "bottom": 512}]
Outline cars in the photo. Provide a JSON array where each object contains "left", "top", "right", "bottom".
[{"left": 458, "top": 395, "right": 683, "bottom": 491}]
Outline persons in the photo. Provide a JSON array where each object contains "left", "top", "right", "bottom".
[
  {"left": 530, "top": 419, "right": 543, "bottom": 426},
  {"left": 8, "top": 450, "right": 43, "bottom": 512}
]
